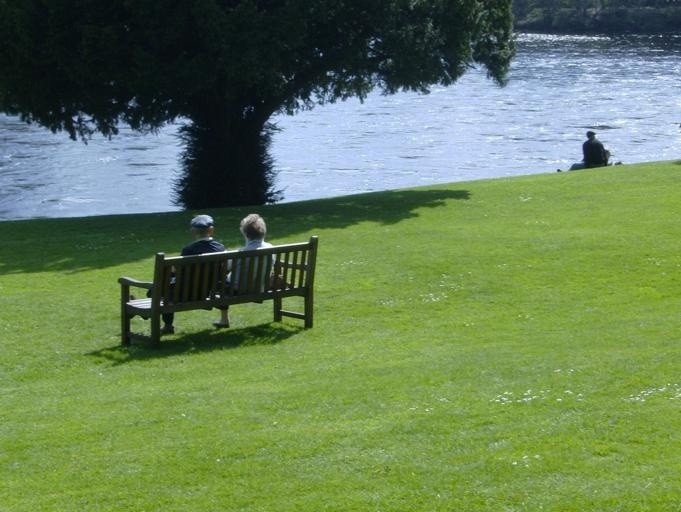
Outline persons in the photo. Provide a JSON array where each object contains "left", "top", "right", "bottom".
[
  {"left": 128, "top": 213, "right": 275, "bottom": 334},
  {"left": 556, "top": 131, "right": 610, "bottom": 172}
]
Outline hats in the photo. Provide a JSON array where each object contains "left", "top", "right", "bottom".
[
  {"left": 586, "top": 131, "right": 597, "bottom": 138},
  {"left": 191, "top": 214, "right": 215, "bottom": 228}
]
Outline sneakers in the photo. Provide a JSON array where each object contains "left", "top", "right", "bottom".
[
  {"left": 212, "top": 320, "right": 230, "bottom": 328},
  {"left": 160, "top": 325, "right": 174, "bottom": 334}
]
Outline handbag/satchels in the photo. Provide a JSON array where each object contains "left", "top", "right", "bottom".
[{"left": 269, "top": 274, "right": 286, "bottom": 289}]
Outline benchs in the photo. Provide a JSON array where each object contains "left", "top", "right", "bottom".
[{"left": 118, "top": 236, "right": 319, "bottom": 353}]
